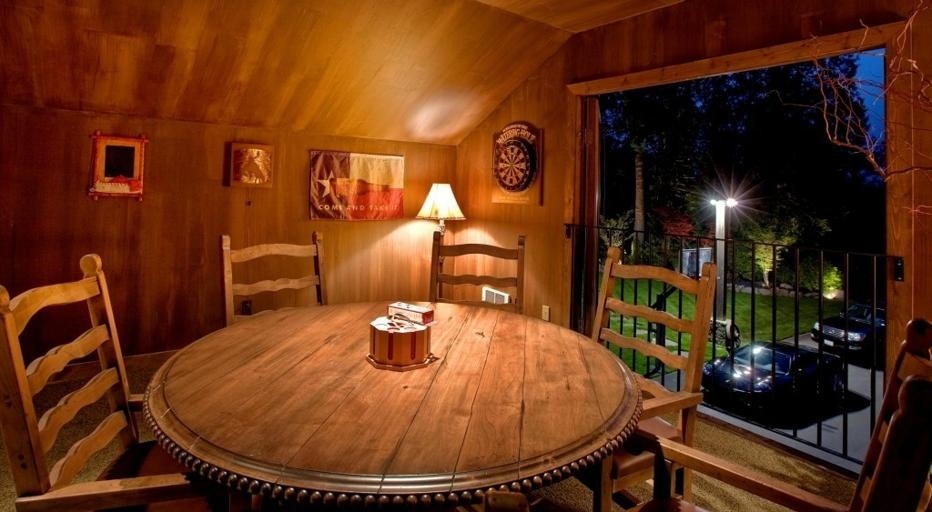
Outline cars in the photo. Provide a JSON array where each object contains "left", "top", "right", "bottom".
[{"left": 805, "top": 300, "right": 893, "bottom": 359}]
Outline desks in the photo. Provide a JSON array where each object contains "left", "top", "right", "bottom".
[{"left": 143, "top": 300, "right": 646, "bottom": 511}]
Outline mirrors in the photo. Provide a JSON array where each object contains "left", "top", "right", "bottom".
[{"left": 86, "top": 128, "right": 151, "bottom": 204}]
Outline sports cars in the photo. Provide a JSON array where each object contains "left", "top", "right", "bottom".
[{"left": 701, "top": 335, "right": 853, "bottom": 417}]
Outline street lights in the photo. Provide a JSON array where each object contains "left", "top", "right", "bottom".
[{"left": 708, "top": 196, "right": 738, "bottom": 330}]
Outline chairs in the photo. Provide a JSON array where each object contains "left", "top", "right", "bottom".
[
  {"left": 218, "top": 230, "right": 331, "bottom": 327},
  {"left": 622, "top": 316, "right": 932, "bottom": 512},
  {"left": 0, "top": 253, "right": 232, "bottom": 512},
  {"left": 571, "top": 245, "right": 718, "bottom": 512},
  {"left": 425, "top": 230, "right": 530, "bottom": 315}
]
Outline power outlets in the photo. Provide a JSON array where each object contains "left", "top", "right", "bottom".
[{"left": 540, "top": 304, "right": 551, "bottom": 322}]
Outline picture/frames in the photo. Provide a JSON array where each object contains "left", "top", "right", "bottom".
[{"left": 227, "top": 141, "right": 280, "bottom": 190}]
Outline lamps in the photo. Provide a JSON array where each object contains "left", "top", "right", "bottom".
[{"left": 413, "top": 182, "right": 468, "bottom": 300}]
